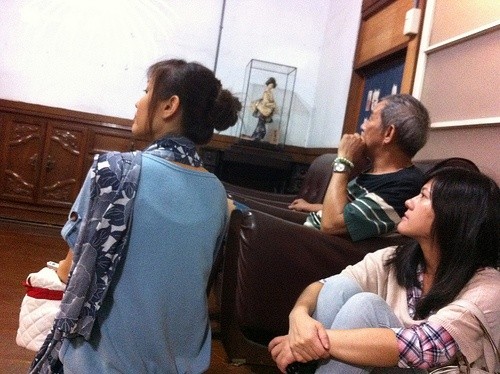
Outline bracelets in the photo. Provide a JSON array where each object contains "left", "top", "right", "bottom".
[{"left": 333, "top": 157, "right": 355, "bottom": 169}]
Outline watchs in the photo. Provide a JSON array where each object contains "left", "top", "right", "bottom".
[{"left": 332, "top": 164, "right": 351, "bottom": 174}]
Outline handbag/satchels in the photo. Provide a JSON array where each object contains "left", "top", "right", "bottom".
[{"left": 15, "top": 267, "right": 68, "bottom": 352}]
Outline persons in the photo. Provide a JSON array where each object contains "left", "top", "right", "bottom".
[
  {"left": 264, "top": 167, "right": 499, "bottom": 374},
  {"left": 223, "top": 90, "right": 433, "bottom": 243},
  {"left": 248, "top": 77, "right": 279, "bottom": 142},
  {"left": 29, "top": 59, "right": 243, "bottom": 373}
]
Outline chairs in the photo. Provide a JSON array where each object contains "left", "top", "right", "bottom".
[
  {"left": 217, "top": 157, "right": 481, "bottom": 364},
  {"left": 206, "top": 153, "right": 336, "bottom": 296}
]
